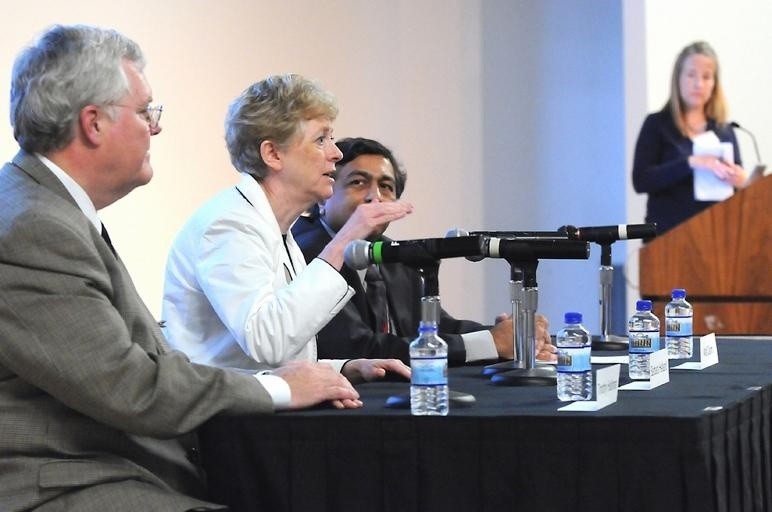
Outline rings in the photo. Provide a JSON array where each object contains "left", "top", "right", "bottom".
[{"left": 716, "top": 159, "right": 720, "bottom": 165}]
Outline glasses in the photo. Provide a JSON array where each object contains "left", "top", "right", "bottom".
[{"left": 106, "top": 100, "right": 163, "bottom": 127}]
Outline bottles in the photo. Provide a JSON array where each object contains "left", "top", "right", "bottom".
[
  {"left": 628, "top": 300, "right": 660, "bottom": 379},
  {"left": 556, "top": 311, "right": 592, "bottom": 401},
  {"left": 409, "top": 322, "right": 449, "bottom": 416},
  {"left": 666, "top": 289, "right": 693, "bottom": 359}
]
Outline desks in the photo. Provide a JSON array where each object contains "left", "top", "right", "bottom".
[{"left": 200, "top": 336, "right": 772, "bottom": 512}]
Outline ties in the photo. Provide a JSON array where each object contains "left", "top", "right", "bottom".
[{"left": 366, "top": 264, "right": 391, "bottom": 335}]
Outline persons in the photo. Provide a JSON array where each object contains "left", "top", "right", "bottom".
[
  {"left": 290, "top": 136, "right": 558, "bottom": 367},
  {"left": 0, "top": 22, "right": 363, "bottom": 512},
  {"left": 161, "top": 75, "right": 412, "bottom": 382},
  {"left": 632, "top": 41, "right": 760, "bottom": 246}
]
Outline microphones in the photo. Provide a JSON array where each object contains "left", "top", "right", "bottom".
[
  {"left": 557, "top": 223, "right": 657, "bottom": 243},
  {"left": 729, "top": 120, "right": 761, "bottom": 165},
  {"left": 444, "top": 228, "right": 560, "bottom": 240},
  {"left": 342, "top": 238, "right": 508, "bottom": 271},
  {"left": 464, "top": 226, "right": 591, "bottom": 261}
]
